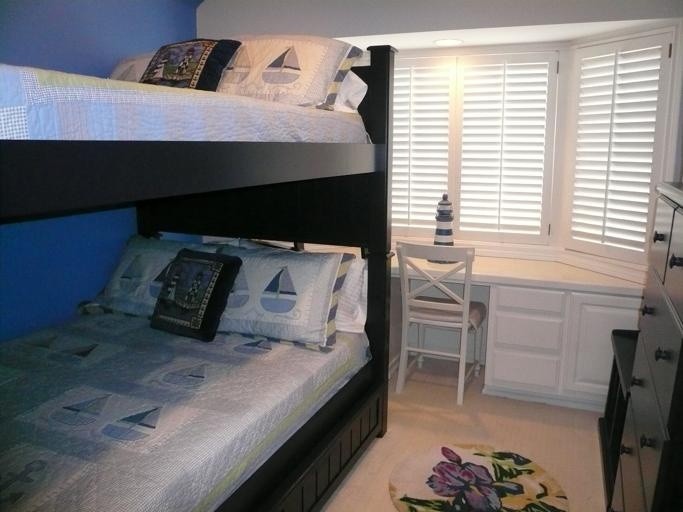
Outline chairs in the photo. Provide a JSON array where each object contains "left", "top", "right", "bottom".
[{"left": 394, "top": 237, "right": 487, "bottom": 406}]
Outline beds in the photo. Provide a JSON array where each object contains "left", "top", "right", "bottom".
[{"left": 0, "top": 42, "right": 400, "bottom": 511}]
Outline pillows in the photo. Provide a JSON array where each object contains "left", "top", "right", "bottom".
[
  {"left": 104, "top": 229, "right": 355, "bottom": 353},
  {"left": 111, "top": 31, "right": 362, "bottom": 112}
]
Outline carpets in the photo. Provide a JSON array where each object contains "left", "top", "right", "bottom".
[{"left": 386, "top": 442, "right": 571, "bottom": 511}]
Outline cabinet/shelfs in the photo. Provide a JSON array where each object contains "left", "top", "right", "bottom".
[
  {"left": 566, "top": 291, "right": 639, "bottom": 409},
  {"left": 601, "top": 181, "right": 683, "bottom": 511},
  {"left": 495, "top": 285, "right": 566, "bottom": 398}
]
